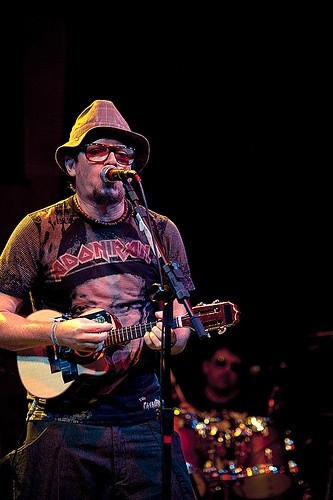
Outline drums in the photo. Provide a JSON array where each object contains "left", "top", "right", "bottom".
[
  {"left": 174, "top": 410, "right": 213, "bottom": 496},
  {"left": 232, "top": 416, "right": 298, "bottom": 498}
]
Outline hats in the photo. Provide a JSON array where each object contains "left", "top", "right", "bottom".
[{"left": 55, "top": 100, "right": 151, "bottom": 177}]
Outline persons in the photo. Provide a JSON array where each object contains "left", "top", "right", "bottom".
[
  {"left": 0, "top": 100, "right": 196, "bottom": 500},
  {"left": 173, "top": 346, "right": 289, "bottom": 500}
]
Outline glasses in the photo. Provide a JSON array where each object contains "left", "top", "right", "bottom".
[{"left": 80, "top": 143, "right": 136, "bottom": 167}]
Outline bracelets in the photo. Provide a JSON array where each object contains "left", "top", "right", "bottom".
[
  {"left": 162, "top": 338, "right": 179, "bottom": 356},
  {"left": 51, "top": 323, "right": 60, "bottom": 346}
]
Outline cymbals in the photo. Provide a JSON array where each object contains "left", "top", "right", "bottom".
[{"left": 306, "top": 332, "right": 333, "bottom": 350}]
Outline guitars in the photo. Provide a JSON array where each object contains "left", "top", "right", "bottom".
[{"left": 17, "top": 299, "right": 240, "bottom": 398}]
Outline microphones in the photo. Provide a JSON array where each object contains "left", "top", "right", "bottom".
[{"left": 100, "top": 165, "right": 137, "bottom": 182}]
